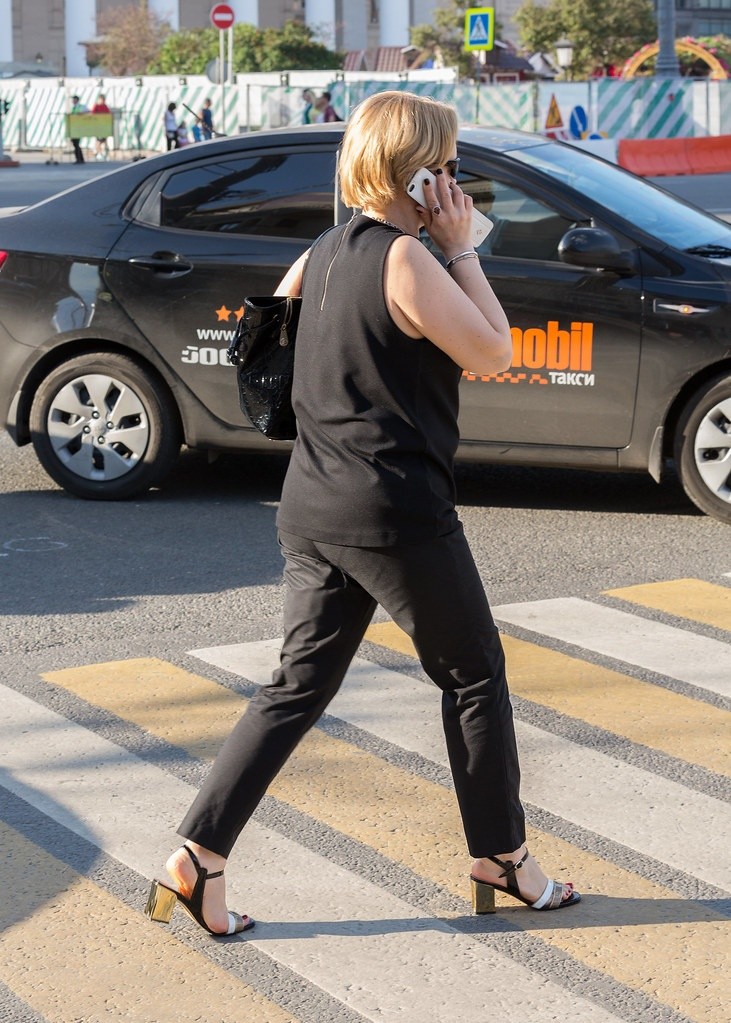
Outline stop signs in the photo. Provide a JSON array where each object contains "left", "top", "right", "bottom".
[{"left": 211, "top": 4, "right": 237, "bottom": 30}]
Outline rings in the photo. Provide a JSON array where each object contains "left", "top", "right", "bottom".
[{"left": 431, "top": 205, "right": 442, "bottom": 216}]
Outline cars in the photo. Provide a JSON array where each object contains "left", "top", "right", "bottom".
[{"left": 1, "top": 121, "right": 731, "bottom": 528}]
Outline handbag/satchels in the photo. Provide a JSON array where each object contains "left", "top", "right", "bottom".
[{"left": 226, "top": 224, "right": 340, "bottom": 441}]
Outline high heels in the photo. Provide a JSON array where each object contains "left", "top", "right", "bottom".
[
  {"left": 144, "top": 845, "right": 255, "bottom": 937},
  {"left": 470, "top": 845, "right": 581, "bottom": 915}
]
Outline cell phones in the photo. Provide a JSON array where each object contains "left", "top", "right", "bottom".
[{"left": 407, "top": 167, "right": 494, "bottom": 248}]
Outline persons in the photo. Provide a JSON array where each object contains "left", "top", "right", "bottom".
[
  {"left": 301, "top": 89, "right": 336, "bottom": 124},
  {"left": 163, "top": 98, "right": 213, "bottom": 151},
  {"left": 144, "top": 90, "right": 581, "bottom": 937},
  {"left": 464, "top": 177, "right": 575, "bottom": 256},
  {"left": 70, "top": 94, "right": 111, "bottom": 164}
]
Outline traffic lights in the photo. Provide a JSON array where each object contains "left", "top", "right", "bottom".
[{"left": 3, "top": 100, "right": 11, "bottom": 115}]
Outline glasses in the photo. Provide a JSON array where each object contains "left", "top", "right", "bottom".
[{"left": 444, "top": 158, "right": 462, "bottom": 179}]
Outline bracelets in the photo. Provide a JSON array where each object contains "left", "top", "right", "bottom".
[{"left": 445, "top": 251, "right": 479, "bottom": 271}]
